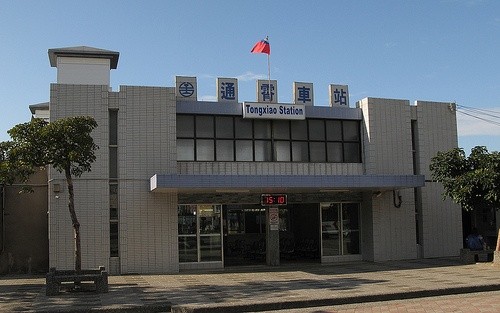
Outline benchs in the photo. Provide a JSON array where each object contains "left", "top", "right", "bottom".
[{"left": 460, "top": 248, "right": 492, "bottom": 264}]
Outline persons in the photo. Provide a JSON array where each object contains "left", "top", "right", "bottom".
[{"left": 465, "top": 225, "right": 485, "bottom": 250}]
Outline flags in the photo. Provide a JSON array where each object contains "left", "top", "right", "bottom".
[{"left": 250, "top": 36, "right": 271, "bottom": 55}]
